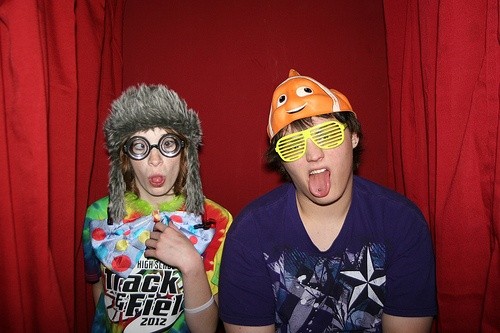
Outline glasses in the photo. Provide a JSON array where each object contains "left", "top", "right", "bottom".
[
  {"left": 124, "top": 133, "right": 186, "bottom": 162},
  {"left": 273, "top": 120, "right": 349, "bottom": 162}
]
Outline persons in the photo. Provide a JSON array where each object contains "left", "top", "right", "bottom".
[
  {"left": 82, "top": 84, "right": 233, "bottom": 333},
  {"left": 218, "top": 69, "right": 436, "bottom": 333}
]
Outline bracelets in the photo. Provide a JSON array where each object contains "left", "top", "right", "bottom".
[{"left": 183, "top": 294, "right": 214, "bottom": 314}]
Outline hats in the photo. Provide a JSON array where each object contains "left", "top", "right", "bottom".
[
  {"left": 265, "top": 68, "right": 357, "bottom": 144},
  {"left": 102, "top": 82, "right": 206, "bottom": 221}
]
